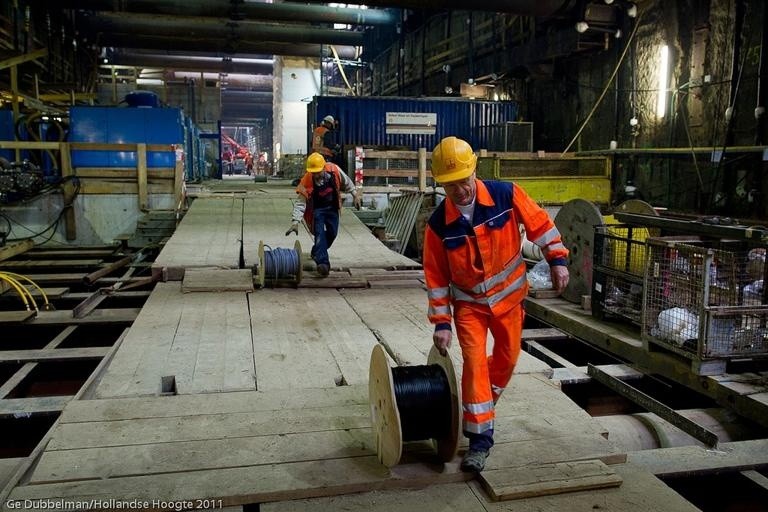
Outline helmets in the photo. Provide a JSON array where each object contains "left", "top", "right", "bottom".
[
  {"left": 430, "top": 136, "right": 478, "bottom": 183},
  {"left": 324, "top": 115, "right": 334, "bottom": 124},
  {"left": 306, "top": 153, "right": 325, "bottom": 172}
]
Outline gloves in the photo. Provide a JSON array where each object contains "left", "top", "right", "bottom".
[
  {"left": 352, "top": 190, "right": 360, "bottom": 211},
  {"left": 286, "top": 220, "right": 299, "bottom": 236}
]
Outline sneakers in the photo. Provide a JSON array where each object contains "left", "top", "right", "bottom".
[{"left": 459, "top": 449, "right": 489, "bottom": 472}]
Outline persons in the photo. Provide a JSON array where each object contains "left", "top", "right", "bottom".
[
  {"left": 283, "top": 151, "right": 362, "bottom": 276},
  {"left": 312, "top": 114, "right": 341, "bottom": 164},
  {"left": 221, "top": 148, "right": 268, "bottom": 177},
  {"left": 422, "top": 136, "right": 571, "bottom": 473}
]
